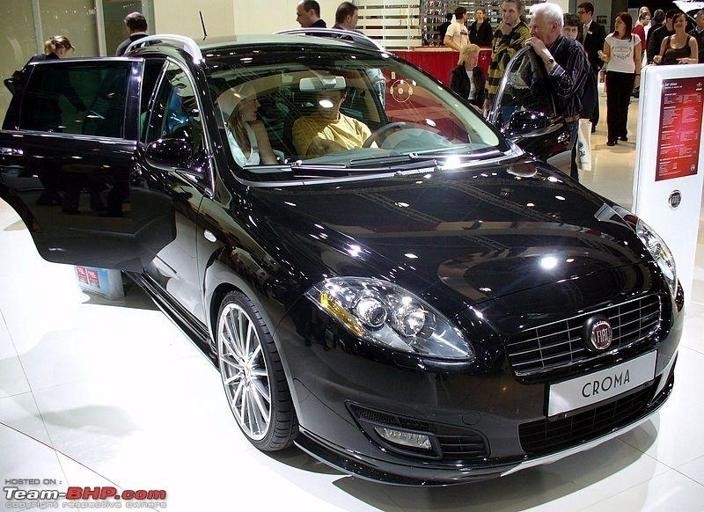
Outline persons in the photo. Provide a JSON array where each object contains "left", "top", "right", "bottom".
[
  {"left": 331, "top": 1, "right": 365, "bottom": 38},
  {"left": 293, "top": 0, "right": 331, "bottom": 37},
  {"left": 4, "top": 33, "right": 88, "bottom": 130},
  {"left": 218, "top": 83, "right": 279, "bottom": 168},
  {"left": 115, "top": 11, "right": 150, "bottom": 56},
  {"left": 291, "top": 80, "right": 379, "bottom": 159}
]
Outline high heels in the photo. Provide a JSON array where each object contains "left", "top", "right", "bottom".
[{"left": 607, "top": 136, "right": 628, "bottom": 147}]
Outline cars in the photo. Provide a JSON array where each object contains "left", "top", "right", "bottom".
[{"left": 0, "top": 27, "right": 683, "bottom": 491}]
